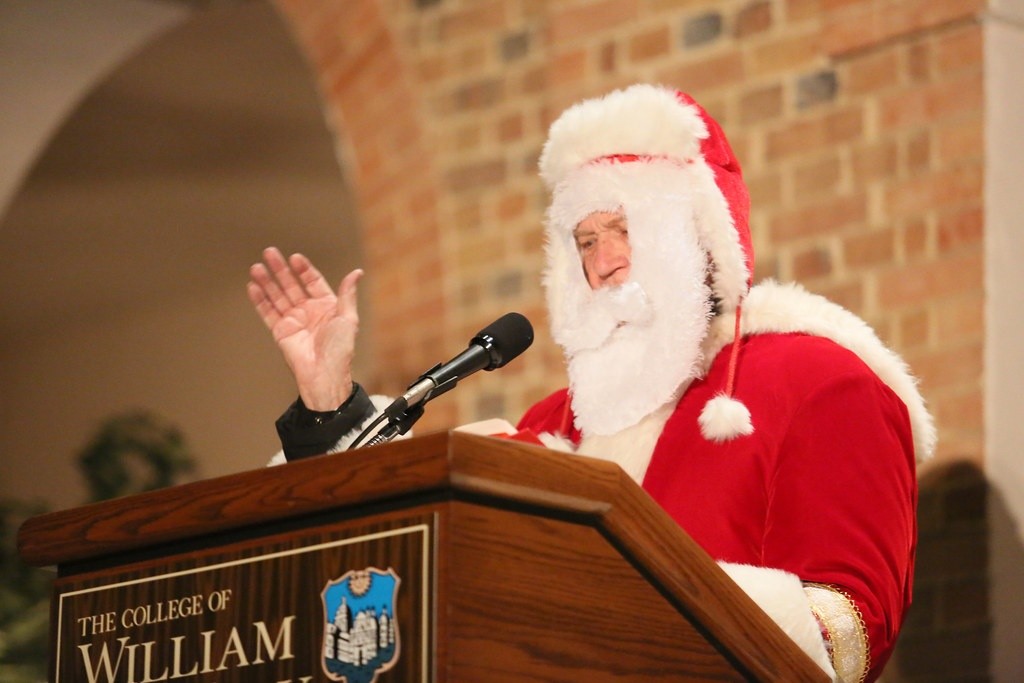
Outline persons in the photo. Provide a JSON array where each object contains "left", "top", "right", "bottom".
[{"left": 243, "top": 82, "right": 937, "bottom": 683}]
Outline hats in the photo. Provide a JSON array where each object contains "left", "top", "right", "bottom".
[{"left": 539, "top": 82, "right": 753, "bottom": 441}]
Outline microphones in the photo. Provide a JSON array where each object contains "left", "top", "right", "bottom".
[{"left": 383, "top": 312, "right": 534, "bottom": 417}]
